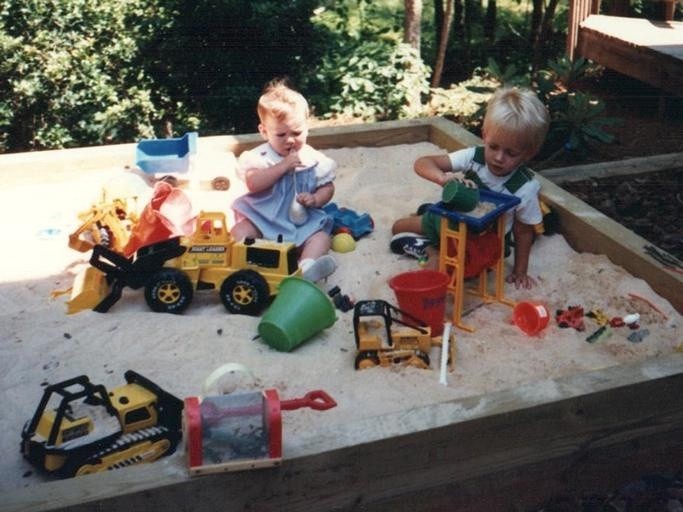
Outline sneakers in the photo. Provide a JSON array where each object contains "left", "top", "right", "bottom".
[{"left": 389, "top": 232, "right": 433, "bottom": 262}]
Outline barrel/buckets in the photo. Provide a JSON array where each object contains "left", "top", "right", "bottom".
[
  {"left": 258, "top": 277, "right": 339, "bottom": 353},
  {"left": 389, "top": 270, "right": 452, "bottom": 338}
]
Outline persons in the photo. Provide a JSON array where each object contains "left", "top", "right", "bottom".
[
  {"left": 390, "top": 87, "right": 543, "bottom": 288},
  {"left": 230, "top": 86, "right": 337, "bottom": 283}
]
